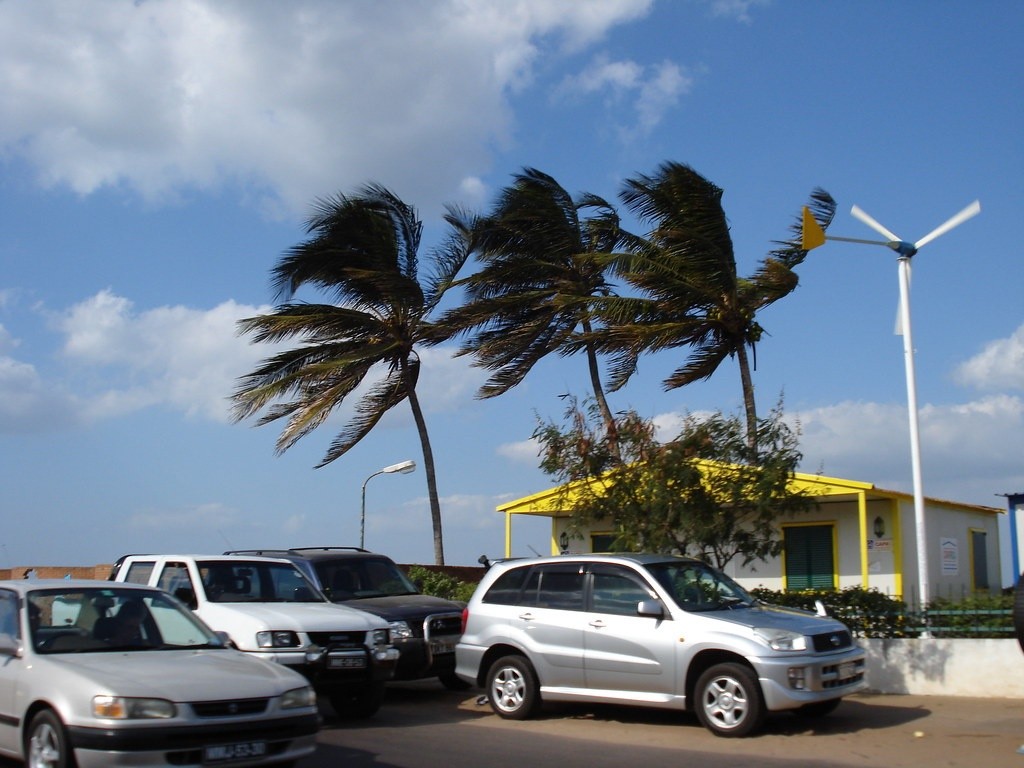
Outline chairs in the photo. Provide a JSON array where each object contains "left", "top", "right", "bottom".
[
  {"left": 92, "top": 617, "right": 116, "bottom": 641},
  {"left": 175, "top": 588, "right": 198, "bottom": 610}
]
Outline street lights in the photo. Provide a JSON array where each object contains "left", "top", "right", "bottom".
[{"left": 361, "top": 459, "right": 419, "bottom": 548}]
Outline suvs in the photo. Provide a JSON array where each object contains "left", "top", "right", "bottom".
[
  {"left": 203, "top": 546, "right": 476, "bottom": 694},
  {"left": 454, "top": 552, "right": 865, "bottom": 739}
]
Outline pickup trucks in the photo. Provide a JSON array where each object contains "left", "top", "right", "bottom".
[{"left": 51, "top": 552, "right": 400, "bottom": 728}]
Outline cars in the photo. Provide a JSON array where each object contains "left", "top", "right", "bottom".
[{"left": 1, "top": 579, "right": 322, "bottom": 768}]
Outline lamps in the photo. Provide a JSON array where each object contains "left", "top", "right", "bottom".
[
  {"left": 560, "top": 532, "right": 568, "bottom": 550},
  {"left": 873, "top": 516, "right": 885, "bottom": 538}
]
